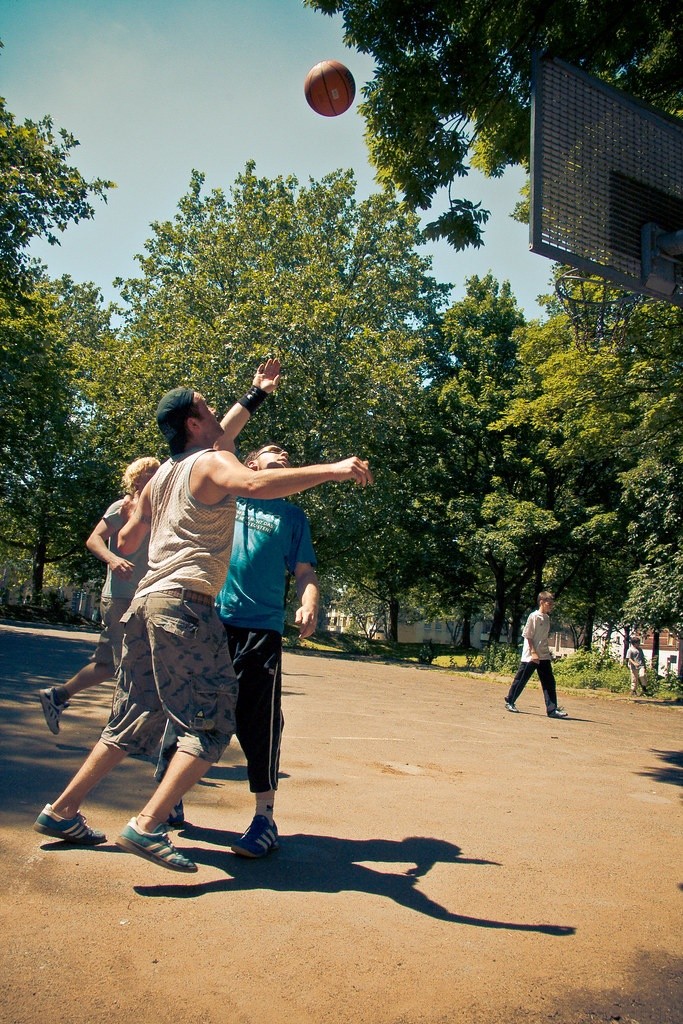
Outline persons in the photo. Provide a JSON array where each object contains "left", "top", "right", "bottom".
[
  {"left": 164, "top": 359, "right": 320, "bottom": 859},
  {"left": 626, "top": 637, "right": 649, "bottom": 694},
  {"left": 40, "top": 458, "right": 162, "bottom": 735},
  {"left": 34, "top": 388, "right": 374, "bottom": 875},
  {"left": 504, "top": 590, "right": 569, "bottom": 718}
]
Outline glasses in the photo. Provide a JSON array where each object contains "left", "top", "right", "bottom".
[{"left": 253, "top": 446, "right": 282, "bottom": 460}]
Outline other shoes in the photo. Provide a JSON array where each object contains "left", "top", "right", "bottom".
[{"left": 642, "top": 686, "right": 648, "bottom": 692}]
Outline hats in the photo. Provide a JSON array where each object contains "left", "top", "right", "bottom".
[{"left": 156, "top": 388, "right": 193, "bottom": 455}]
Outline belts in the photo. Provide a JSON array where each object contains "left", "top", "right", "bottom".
[{"left": 158, "top": 588, "right": 215, "bottom": 608}]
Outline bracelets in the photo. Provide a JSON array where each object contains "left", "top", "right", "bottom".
[{"left": 238, "top": 385, "right": 269, "bottom": 417}]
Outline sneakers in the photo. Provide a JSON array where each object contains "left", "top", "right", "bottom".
[
  {"left": 33, "top": 803, "right": 106, "bottom": 845},
  {"left": 115, "top": 817, "right": 198, "bottom": 873},
  {"left": 231, "top": 814, "right": 279, "bottom": 857},
  {"left": 505, "top": 703, "right": 518, "bottom": 713},
  {"left": 549, "top": 707, "right": 567, "bottom": 718},
  {"left": 40, "top": 687, "right": 70, "bottom": 735},
  {"left": 154, "top": 766, "right": 184, "bottom": 826}
]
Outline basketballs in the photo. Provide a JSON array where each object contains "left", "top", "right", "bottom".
[{"left": 304, "top": 58, "right": 356, "bottom": 118}]
